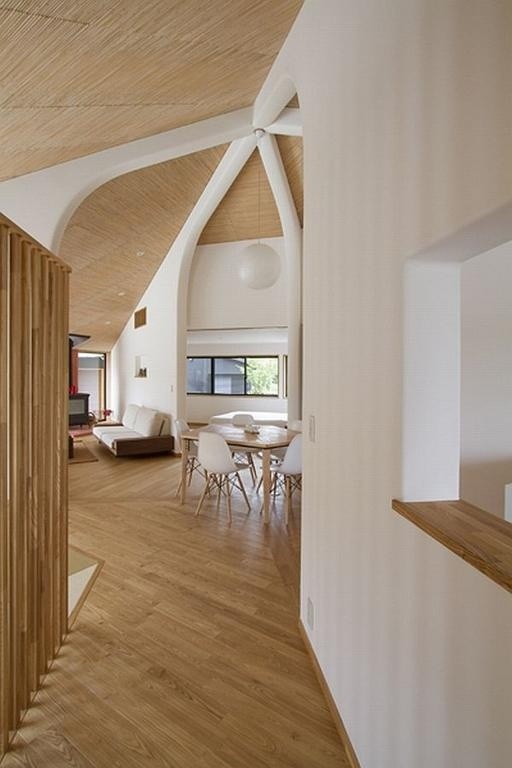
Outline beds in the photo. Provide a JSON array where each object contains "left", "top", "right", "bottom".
[{"left": 209, "top": 412, "right": 287, "bottom": 427}]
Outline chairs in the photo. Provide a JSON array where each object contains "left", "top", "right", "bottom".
[{"left": 174, "top": 415, "right": 302, "bottom": 526}]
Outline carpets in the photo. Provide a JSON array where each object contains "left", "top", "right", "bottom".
[{"left": 67, "top": 440, "right": 99, "bottom": 464}]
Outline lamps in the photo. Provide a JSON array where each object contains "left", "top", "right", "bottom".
[{"left": 234, "top": 129, "right": 282, "bottom": 289}]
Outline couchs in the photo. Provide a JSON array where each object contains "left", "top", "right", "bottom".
[{"left": 91, "top": 404, "right": 173, "bottom": 457}]
